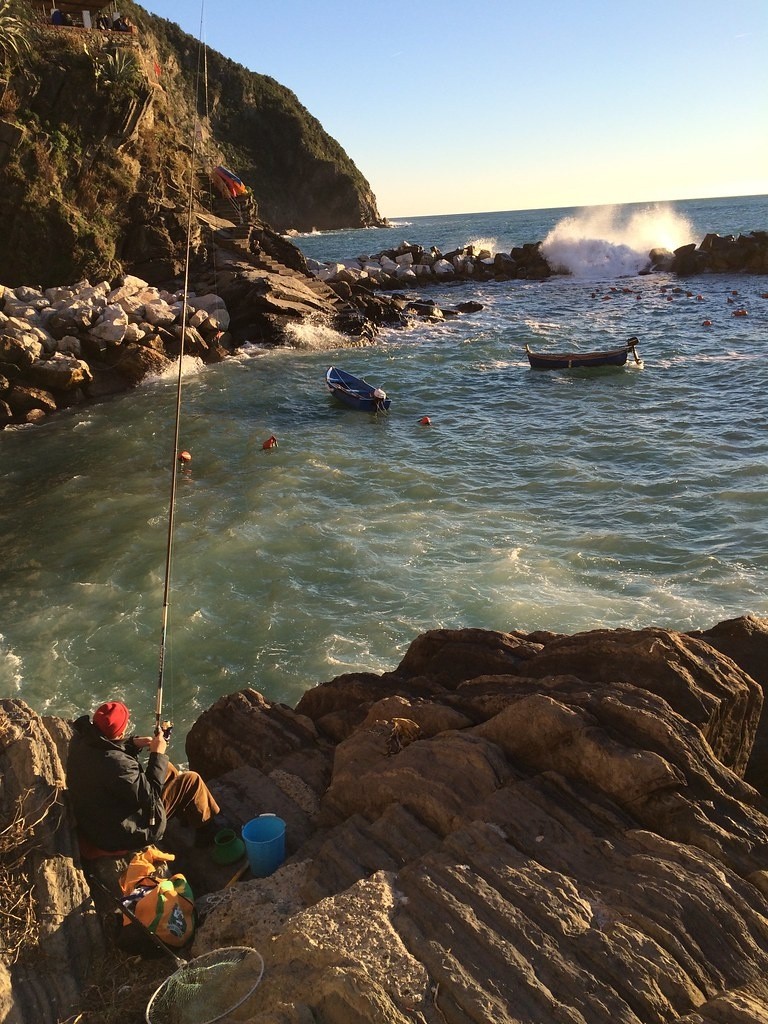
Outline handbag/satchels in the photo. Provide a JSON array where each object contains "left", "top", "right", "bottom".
[{"left": 120, "top": 844, "right": 196, "bottom": 948}]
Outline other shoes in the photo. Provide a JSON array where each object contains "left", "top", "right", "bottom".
[{"left": 196, "top": 823, "right": 234, "bottom": 847}]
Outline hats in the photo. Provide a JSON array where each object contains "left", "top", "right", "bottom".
[{"left": 93, "top": 701, "right": 129, "bottom": 738}]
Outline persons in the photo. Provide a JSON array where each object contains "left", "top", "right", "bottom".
[
  {"left": 66, "top": 701, "right": 243, "bottom": 853},
  {"left": 51, "top": 8, "right": 132, "bottom": 31}
]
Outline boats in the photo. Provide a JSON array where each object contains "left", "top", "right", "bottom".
[
  {"left": 325, "top": 365, "right": 392, "bottom": 412},
  {"left": 523, "top": 338, "right": 639, "bottom": 369}
]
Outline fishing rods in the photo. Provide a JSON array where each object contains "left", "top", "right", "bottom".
[{"left": 152, "top": 0, "right": 207, "bottom": 739}]
